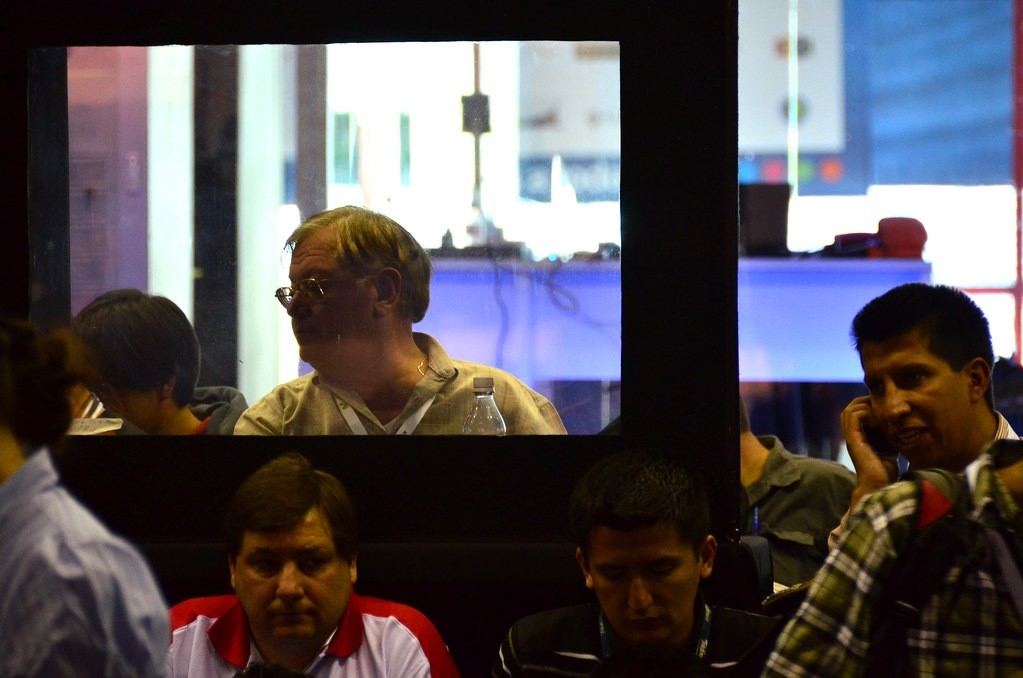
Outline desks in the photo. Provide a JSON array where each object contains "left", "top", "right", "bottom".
[{"left": 292, "top": 254, "right": 935, "bottom": 457}]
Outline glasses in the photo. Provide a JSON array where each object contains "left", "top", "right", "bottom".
[{"left": 274, "top": 275, "right": 368, "bottom": 310}]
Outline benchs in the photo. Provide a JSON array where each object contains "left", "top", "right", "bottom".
[{"left": 126, "top": 532, "right": 773, "bottom": 678}]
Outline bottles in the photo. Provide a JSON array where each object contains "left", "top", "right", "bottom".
[{"left": 460, "top": 376, "right": 508, "bottom": 435}]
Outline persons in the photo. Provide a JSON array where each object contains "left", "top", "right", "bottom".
[
  {"left": 0, "top": 320, "right": 171, "bottom": 678},
  {"left": 761, "top": 438, "right": 1023, "bottom": 678},
  {"left": 737, "top": 391, "right": 858, "bottom": 593},
  {"left": 72, "top": 289, "right": 247, "bottom": 435},
  {"left": 165, "top": 454, "right": 460, "bottom": 678},
  {"left": 489, "top": 449, "right": 784, "bottom": 678},
  {"left": 233, "top": 210, "right": 569, "bottom": 436},
  {"left": 827, "top": 283, "right": 1023, "bottom": 553}
]
